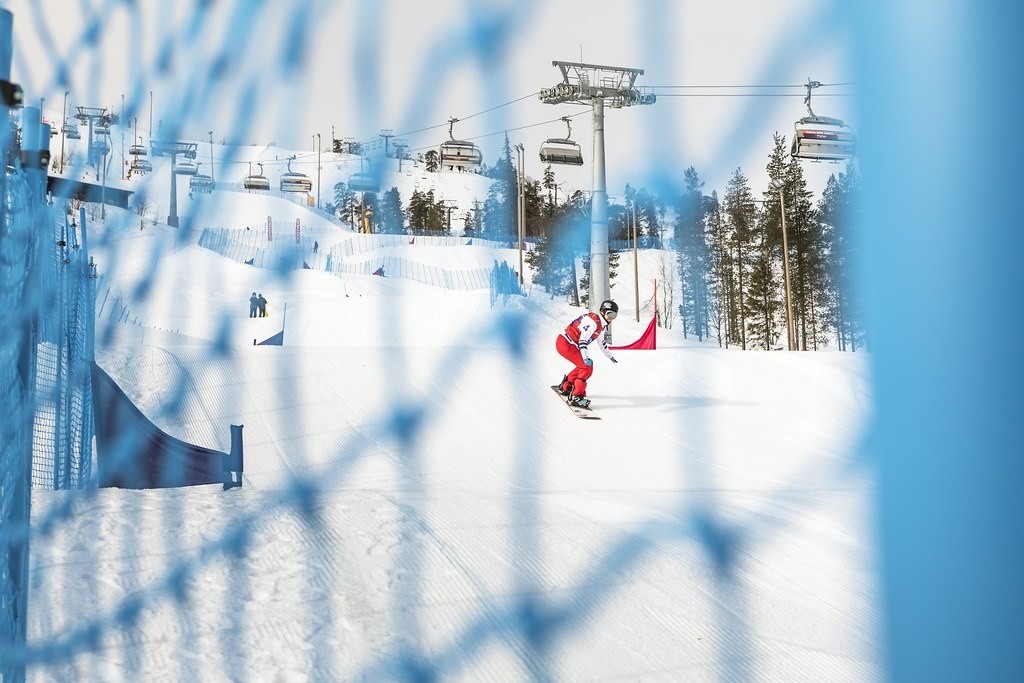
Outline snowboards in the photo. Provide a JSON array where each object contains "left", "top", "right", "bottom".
[{"left": 552, "top": 385, "right": 601, "bottom": 420}]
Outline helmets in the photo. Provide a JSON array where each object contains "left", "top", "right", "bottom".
[{"left": 600, "top": 300, "right": 619, "bottom": 313}]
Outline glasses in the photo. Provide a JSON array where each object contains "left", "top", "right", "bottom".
[{"left": 605, "top": 310, "right": 617, "bottom": 319}]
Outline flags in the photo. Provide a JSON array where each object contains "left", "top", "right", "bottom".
[{"left": 608, "top": 317, "right": 656, "bottom": 350}]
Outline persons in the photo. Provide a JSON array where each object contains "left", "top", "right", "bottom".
[
  {"left": 379, "top": 266, "right": 385, "bottom": 276},
  {"left": 258, "top": 294, "right": 267, "bottom": 317},
  {"left": 314, "top": 241, "right": 319, "bottom": 253},
  {"left": 249, "top": 292, "right": 258, "bottom": 318},
  {"left": 556, "top": 300, "right": 618, "bottom": 407}
]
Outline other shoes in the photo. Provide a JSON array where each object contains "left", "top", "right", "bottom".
[
  {"left": 567, "top": 385, "right": 591, "bottom": 407},
  {"left": 558, "top": 374, "right": 574, "bottom": 396}
]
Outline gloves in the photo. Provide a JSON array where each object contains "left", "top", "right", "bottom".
[
  {"left": 610, "top": 357, "right": 618, "bottom": 363},
  {"left": 583, "top": 358, "right": 593, "bottom": 365}
]
